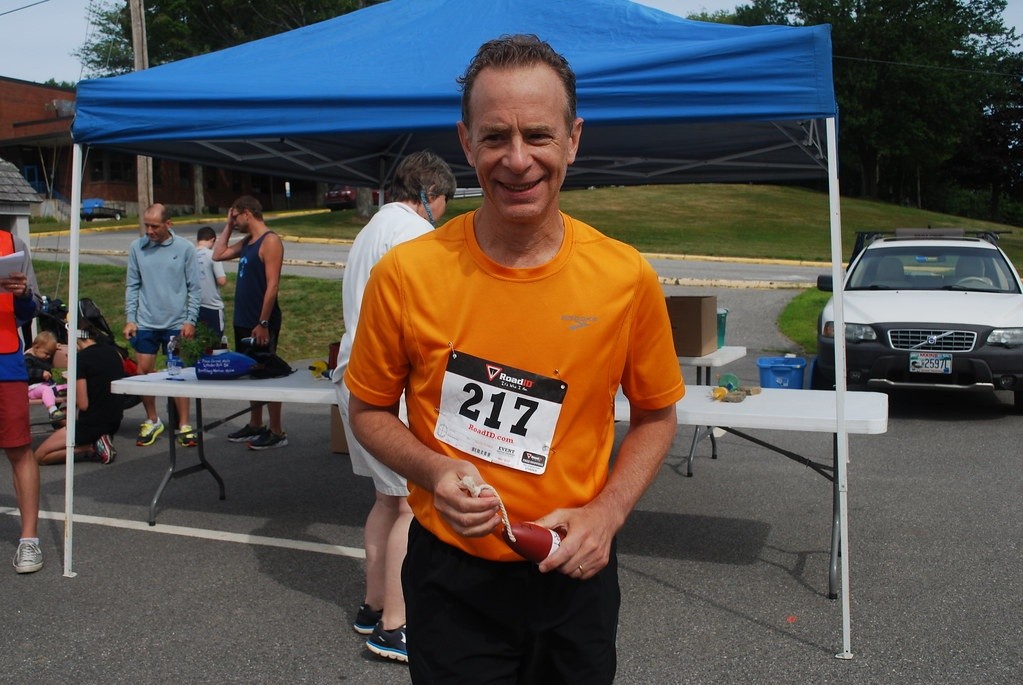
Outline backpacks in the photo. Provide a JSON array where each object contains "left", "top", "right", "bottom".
[{"left": 37, "top": 296, "right": 114, "bottom": 349}]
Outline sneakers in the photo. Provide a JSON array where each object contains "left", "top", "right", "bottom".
[
  {"left": 178, "top": 426, "right": 199, "bottom": 447},
  {"left": 246, "top": 429, "right": 288, "bottom": 450},
  {"left": 96, "top": 434, "right": 117, "bottom": 464},
  {"left": 226, "top": 422, "right": 268, "bottom": 442},
  {"left": 13, "top": 540, "right": 45, "bottom": 573},
  {"left": 136, "top": 417, "right": 165, "bottom": 446},
  {"left": 366, "top": 621, "right": 408, "bottom": 662},
  {"left": 353, "top": 601, "right": 384, "bottom": 634}
]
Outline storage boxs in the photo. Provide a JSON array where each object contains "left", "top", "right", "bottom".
[
  {"left": 330, "top": 404, "right": 350, "bottom": 453},
  {"left": 665, "top": 296, "right": 719, "bottom": 356},
  {"left": 756, "top": 355, "right": 806, "bottom": 389}
]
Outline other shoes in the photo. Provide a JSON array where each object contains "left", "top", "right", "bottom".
[{"left": 49, "top": 410, "right": 65, "bottom": 422}]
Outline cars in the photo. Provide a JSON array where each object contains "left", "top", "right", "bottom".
[{"left": 323, "top": 183, "right": 388, "bottom": 212}]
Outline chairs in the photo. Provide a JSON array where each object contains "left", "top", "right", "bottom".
[
  {"left": 21, "top": 317, "right": 67, "bottom": 426},
  {"left": 955, "top": 256, "right": 994, "bottom": 288},
  {"left": 877, "top": 257, "right": 912, "bottom": 287}
]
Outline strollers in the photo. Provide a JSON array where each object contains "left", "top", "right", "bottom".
[{"left": 13, "top": 292, "right": 69, "bottom": 431}]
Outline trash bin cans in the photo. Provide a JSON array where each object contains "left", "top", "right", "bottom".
[{"left": 756, "top": 356, "right": 807, "bottom": 389}]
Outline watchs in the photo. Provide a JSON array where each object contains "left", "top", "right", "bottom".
[{"left": 258, "top": 320, "right": 269, "bottom": 328}]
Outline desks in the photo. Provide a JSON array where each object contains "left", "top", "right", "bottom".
[
  {"left": 668, "top": 345, "right": 748, "bottom": 477},
  {"left": 112, "top": 367, "right": 888, "bottom": 598}
]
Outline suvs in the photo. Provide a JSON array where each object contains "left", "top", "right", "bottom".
[{"left": 810, "top": 221, "right": 1022, "bottom": 415}]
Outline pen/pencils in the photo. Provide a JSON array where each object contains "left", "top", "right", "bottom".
[{"left": 166, "top": 378, "right": 184, "bottom": 381}]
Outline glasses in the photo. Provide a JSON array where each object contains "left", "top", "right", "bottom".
[{"left": 232, "top": 211, "right": 244, "bottom": 221}]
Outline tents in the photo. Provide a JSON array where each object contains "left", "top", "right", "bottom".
[{"left": 63, "top": 0, "right": 854, "bottom": 660}]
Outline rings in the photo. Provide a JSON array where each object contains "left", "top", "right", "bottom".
[
  {"left": 15, "top": 284, "right": 17, "bottom": 288},
  {"left": 578, "top": 565, "right": 585, "bottom": 576}
]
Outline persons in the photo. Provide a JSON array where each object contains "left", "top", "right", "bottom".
[
  {"left": 23, "top": 331, "right": 66, "bottom": 424},
  {"left": 332, "top": 151, "right": 457, "bottom": 663},
  {"left": 212, "top": 195, "right": 289, "bottom": 450},
  {"left": 122, "top": 204, "right": 202, "bottom": 447},
  {"left": 32, "top": 317, "right": 124, "bottom": 464},
  {"left": 195, "top": 226, "right": 227, "bottom": 350},
  {"left": 0, "top": 230, "right": 44, "bottom": 574},
  {"left": 343, "top": 33, "right": 687, "bottom": 685}
]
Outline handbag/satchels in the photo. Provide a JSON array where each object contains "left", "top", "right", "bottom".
[{"left": 99, "top": 333, "right": 145, "bottom": 410}]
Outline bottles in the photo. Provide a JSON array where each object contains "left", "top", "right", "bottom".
[
  {"left": 41, "top": 296, "right": 48, "bottom": 311},
  {"left": 718, "top": 374, "right": 739, "bottom": 393},
  {"left": 716, "top": 308, "right": 728, "bottom": 348},
  {"left": 309, "top": 360, "right": 328, "bottom": 377},
  {"left": 167, "top": 336, "right": 182, "bottom": 375}
]
